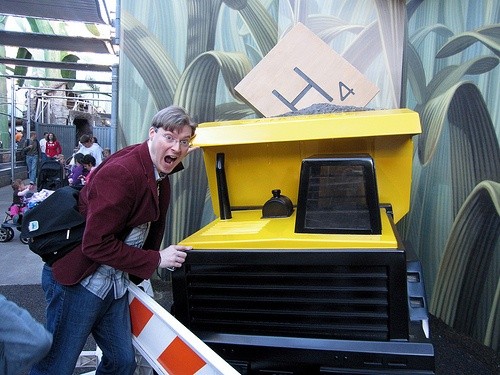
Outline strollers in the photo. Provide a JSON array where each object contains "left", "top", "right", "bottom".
[
  {"left": 36, "top": 156, "right": 73, "bottom": 193},
  {"left": 0, "top": 188, "right": 55, "bottom": 245}
]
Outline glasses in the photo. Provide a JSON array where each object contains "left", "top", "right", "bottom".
[{"left": 155, "top": 127, "right": 191, "bottom": 148}]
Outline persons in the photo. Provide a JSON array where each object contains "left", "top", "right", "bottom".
[
  {"left": 30, "top": 105, "right": 195, "bottom": 375},
  {"left": 23, "top": 132, "right": 111, "bottom": 185},
  {"left": 73, "top": 102, "right": 93, "bottom": 147},
  {"left": 10, "top": 179, "right": 33, "bottom": 224}
]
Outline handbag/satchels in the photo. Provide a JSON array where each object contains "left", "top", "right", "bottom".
[{"left": 21, "top": 186, "right": 87, "bottom": 265}]
